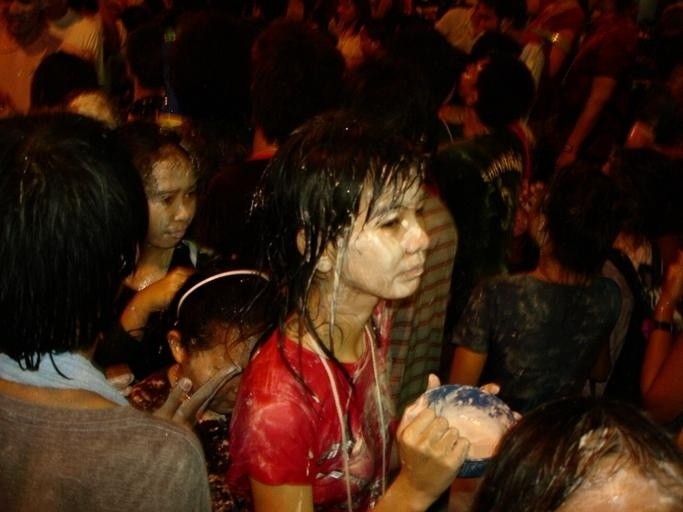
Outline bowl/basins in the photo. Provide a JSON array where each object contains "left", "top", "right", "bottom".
[{"left": 421, "top": 384, "right": 518, "bottom": 480}]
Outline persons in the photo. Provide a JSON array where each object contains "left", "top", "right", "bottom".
[
  {"left": 127, "top": 259, "right": 283, "bottom": 512},
  {"left": 0, "top": 110, "right": 218, "bottom": 512},
  {"left": 224, "top": 107, "right": 527, "bottom": 512},
  {"left": 469, "top": 391, "right": 682, "bottom": 511},
  {"left": 2, "top": 1, "right": 682, "bottom": 488}
]
